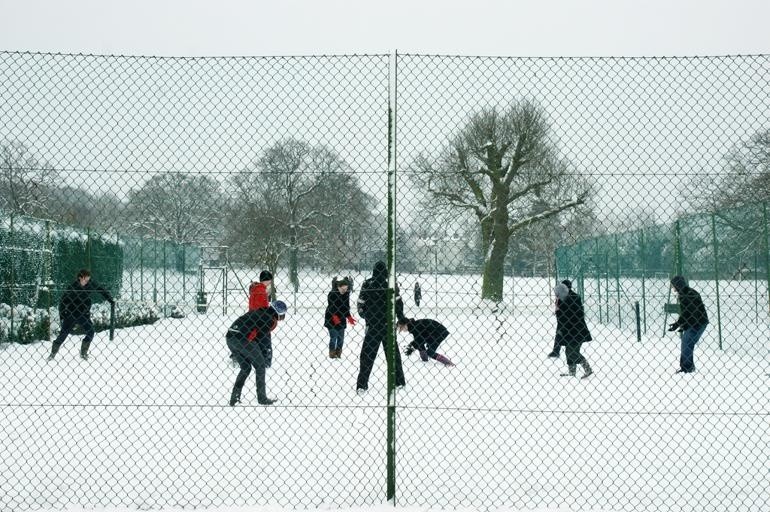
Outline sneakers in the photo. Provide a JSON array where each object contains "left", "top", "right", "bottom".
[
  {"left": 396, "top": 382, "right": 405, "bottom": 387},
  {"left": 230, "top": 398, "right": 242, "bottom": 406},
  {"left": 330, "top": 347, "right": 342, "bottom": 358},
  {"left": 259, "top": 397, "right": 276, "bottom": 405},
  {"left": 676, "top": 363, "right": 694, "bottom": 373},
  {"left": 548, "top": 352, "right": 559, "bottom": 357},
  {"left": 357, "top": 383, "right": 368, "bottom": 392}
]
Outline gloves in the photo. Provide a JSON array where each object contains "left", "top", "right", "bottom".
[
  {"left": 668, "top": 322, "right": 688, "bottom": 332},
  {"left": 264, "top": 358, "right": 271, "bottom": 367},
  {"left": 346, "top": 315, "right": 356, "bottom": 325},
  {"left": 332, "top": 314, "right": 341, "bottom": 324},
  {"left": 402, "top": 344, "right": 413, "bottom": 356}
]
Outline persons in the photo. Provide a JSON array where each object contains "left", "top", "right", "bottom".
[
  {"left": 324, "top": 277, "right": 357, "bottom": 360},
  {"left": 548, "top": 279, "right": 583, "bottom": 357},
  {"left": 230, "top": 270, "right": 273, "bottom": 368},
  {"left": 225, "top": 300, "right": 288, "bottom": 406},
  {"left": 667, "top": 276, "right": 709, "bottom": 373},
  {"left": 396, "top": 316, "right": 456, "bottom": 369},
  {"left": 355, "top": 260, "right": 406, "bottom": 395},
  {"left": 553, "top": 284, "right": 593, "bottom": 379},
  {"left": 413, "top": 282, "right": 422, "bottom": 307},
  {"left": 45, "top": 268, "right": 115, "bottom": 361},
  {"left": 348, "top": 276, "right": 355, "bottom": 293}
]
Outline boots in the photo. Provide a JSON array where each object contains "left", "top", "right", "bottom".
[
  {"left": 47, "top": 341, "right": 60, "bottom": 361},
  {"left": 80, "top": 339, "right": 90, "bottom": 360},
  {"left": 580, "top": 359, "right": 593, "bottom": 379},
  {"left": 560, "top": 363, "right": 576, "bottom": 376},
  {"left": 420, "top": 350, "right": 455, "bottom": 367}
]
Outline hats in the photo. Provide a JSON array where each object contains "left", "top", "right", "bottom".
[
  {"left": 553, "top": 284, "right": 569, "bottom": 301},
  {"left": 270, "top": 300, "right": 287, "bottom": 315},
  {"left": 671, "top": 276, "right": 685, "bottom": 293},
  {"left": 373, "top": 260, "right": 388, "bottom": 279},
  {"left": 260, "top": 270, "right": 273, "bottom": 282}
]
